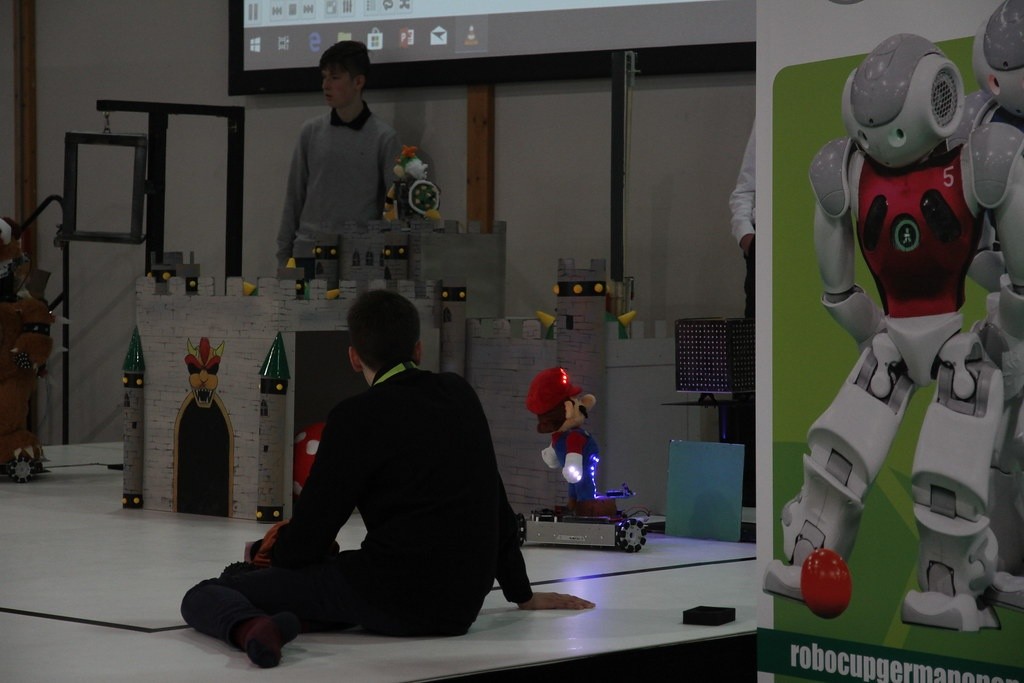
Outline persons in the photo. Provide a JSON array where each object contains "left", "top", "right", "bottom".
[
  {"left": 527, "top": 367, "right": 598, "bottom": 509},
  {"left": 180, "top": 288, "right": 596, "bottom": 670},
  {"left": 275, "top": 40, "right": 401, "bottom": 280},
  {"left": 728, "top": 117, "right": 758, "bottom": 321},
  {"left": 760, "top": 0, "right": 1023, "bottom": 632}
]
